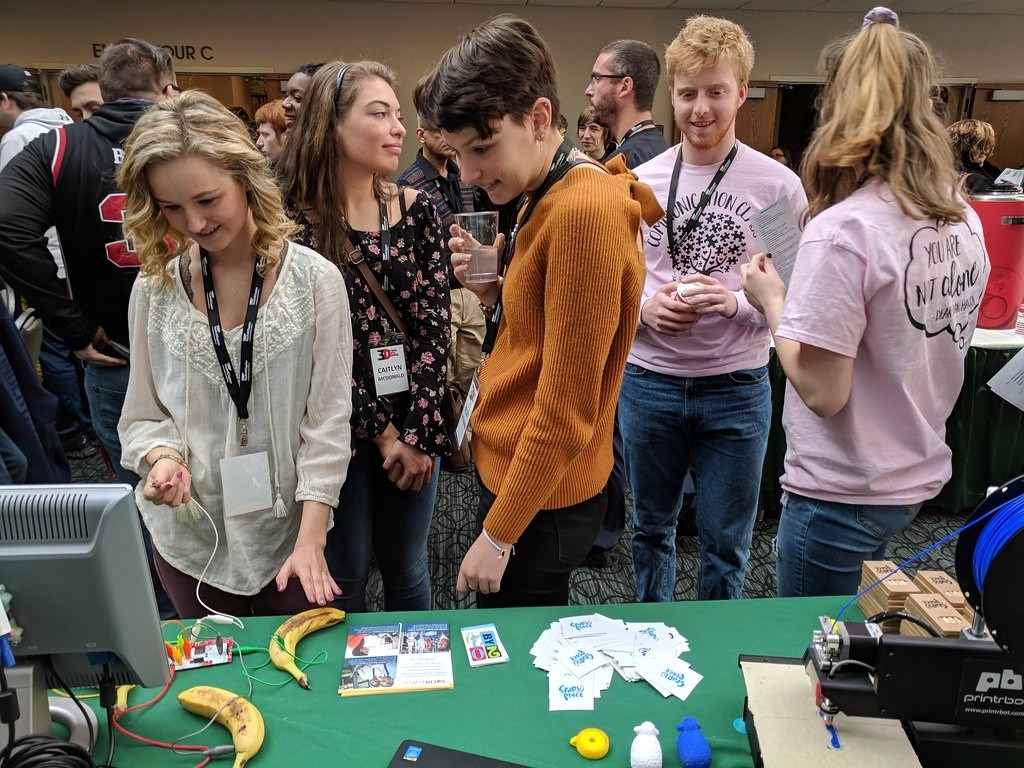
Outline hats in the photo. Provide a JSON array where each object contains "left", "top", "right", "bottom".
[{"left": 0, "top": 63, "right": 41, "bottom": 92}]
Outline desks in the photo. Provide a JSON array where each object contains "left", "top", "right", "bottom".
[{"left": 44, "top": 594, "right": 913, "bottom": 768}]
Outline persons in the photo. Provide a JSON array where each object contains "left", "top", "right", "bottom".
[
  {"left": 348, "top": 634, "right": 370, "bottom": 656},
  {"left": 407, "top": 13, "right": 667, "bottom": 610},
  {"left": 739, "top": 7, "right": 994, "bottom": 596},
  {"left": 272, "top": 60, "right": 458, "bottom": 615},
  {"left": 117, "top": 90, "right": 353, "bottom": 618},
  {"left": 944, "top": 116, "right": 997, "bottom": 195},
  {"left": 559, "top": 115, "right": 568, "bottom": 137},
  {"left": 358, "top": 662, "right": 397, "bottom": 687},
  {"left": 436, "top": 630, "right": 450, "bottom": 651},
  {"left": 770, "top": 146, "right": 793, "bottom": 170},
  {"left": 618, "top": 17, "right": 814, "bottom": 603},
  {"left": 253, "top": 98, "right": 293, "bottom": 162},
  {"left": 281, "top": 62, "right": 327, "bottom": 128},
  {"left": 396, "top": 109, "right": 489, "bottom": 446},
  {"left": 57, "top": 61, "right": 106, "bottom": 123},
  {"left": 1, "top": 63, "right": 101, "bottom": 485},
  {"left": 0, "top": 39, "right": 180, "bottom": 485},
  {"left": 574, "top": 37, "right": 698, "bottom": 569},
  {"left": 578, "top": 106, "right": 617, "bottom": 165},
  {"left": 420, "top": 630, "right": 437, "bottom": 650}
]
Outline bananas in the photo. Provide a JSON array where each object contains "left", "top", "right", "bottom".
[
  {"left": 268, "top": 607, "right": 346, "bottom": 689},
  {"left": 177, "top": 686, "right": 265, "bottom": 768}
]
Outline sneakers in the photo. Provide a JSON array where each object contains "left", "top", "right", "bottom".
[{"left": 67, "top": 424, "right": 101, "bottom": 459}]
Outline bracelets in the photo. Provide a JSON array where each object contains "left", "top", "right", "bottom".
[
  {"left": 151, "top": 454, "right": 193, "bottom": 474},
  {"left": 482, "top": 531, "right": 517, "bottom": 559}
]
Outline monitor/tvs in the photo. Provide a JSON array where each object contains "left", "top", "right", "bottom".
[{"left": 0, "top": 483, "right": 171, "bottom": 766}]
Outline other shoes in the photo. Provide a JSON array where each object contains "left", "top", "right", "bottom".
[{"left": 587, "top": 544, "right": 619, "bottom": 567}]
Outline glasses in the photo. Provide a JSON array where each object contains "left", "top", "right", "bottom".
[{"left": 591, "top": 74, "right": 635, "bottom": 85}]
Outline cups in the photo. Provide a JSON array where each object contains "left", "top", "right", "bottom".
[
  {"left": 453, "top": 210, "right": 499, "bottom": 284},
  {"left": 668, "top": 282, "right": 706, "bottom": 338}
]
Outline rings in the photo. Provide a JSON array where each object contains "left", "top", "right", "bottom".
[{"left": 468, "top": 584, "right": 480, "bottom": 590}]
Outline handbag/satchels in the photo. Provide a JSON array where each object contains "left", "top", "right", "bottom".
[{"left": 440, "top": 384, "right": 471, "bottom": 473}]
